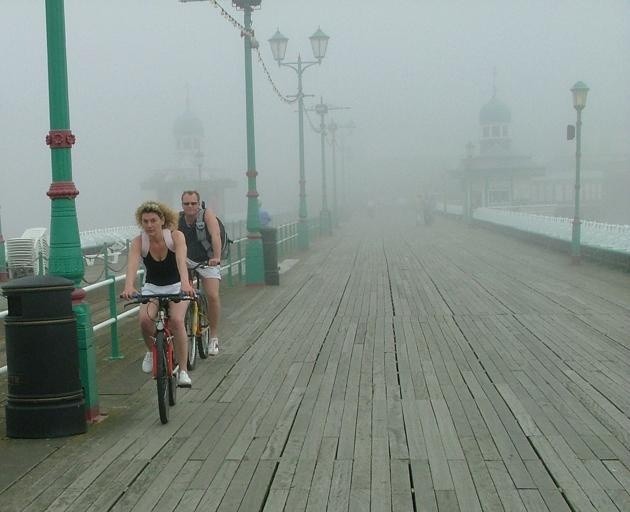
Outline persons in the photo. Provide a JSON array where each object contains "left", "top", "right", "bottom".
[
  {"left": 119, "top": 198, "right": 203, "bottom": 387},
  {"left": 173, "top": 189, "right": 228, "bottom": 357}
]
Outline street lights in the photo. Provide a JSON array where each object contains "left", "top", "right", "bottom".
[
  {"left": 568, "top": 78, "right": 591, "bottom": 261},
  {"left": 267, "top": 20, "right": 332, "bottom": 261},
  {"left": 464, "top": 140, "right": 474, "bottom": 231},
  {"left": 190, "top": 146, "right": 208, "bottom": 182}
]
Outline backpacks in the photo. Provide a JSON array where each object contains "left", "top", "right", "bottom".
[{"left": 196, "top": 200, "right": 233, "bottom": 259}]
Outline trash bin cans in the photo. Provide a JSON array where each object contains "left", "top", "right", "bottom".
[
  {"left": 2, "top": 275, "right": 89, "bottom": 439},
  {"left": 261, "top": 228, "right": 279, "bottom": 286}
]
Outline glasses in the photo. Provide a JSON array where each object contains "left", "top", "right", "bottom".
[{"left": 184, "top": 202, "right": 197, "bottom": 205}]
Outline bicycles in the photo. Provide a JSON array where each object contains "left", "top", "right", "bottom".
[
  {"left": 183, "top": 260, "right": 209, "bottom": 371},
  {"left": 117, "top": 292, "right": 203, "bottom": 425}
]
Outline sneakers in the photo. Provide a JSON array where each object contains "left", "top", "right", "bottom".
[
  {"left": 180, "top": 371, "right": 191, "bottom": 386},
  {"left": 208, "top": 338, "right": 219, "bottom": 355},
  {"left": 142, "top": 351, "right": 153, "bottom": 373}
]
